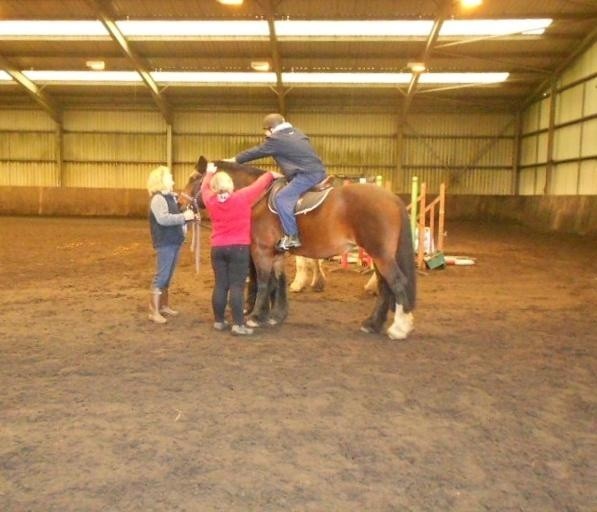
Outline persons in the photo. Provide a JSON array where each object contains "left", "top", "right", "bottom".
[
  {"left": 200, "top": 161, "right": 284, "bottom": 336},
  {"left": 146, "top": 164, "right": 196, "bottom": 324},
  {"left": 222, "top": 111, "right": 330, "bottom": 253}
]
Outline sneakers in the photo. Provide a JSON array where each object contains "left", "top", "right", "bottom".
[
  {"left": 274, "top": 234, "right": 301, "bottom": 252},
  {"left": 213, "top": 320, "right": 228, "bottom": 330},
  {"left": 231, "top": 324, "right": 253, "bottom": 336}
]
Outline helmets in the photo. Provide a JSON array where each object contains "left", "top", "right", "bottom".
[{"left": 262, "top": 113, "right": 284, "bottom": 130}]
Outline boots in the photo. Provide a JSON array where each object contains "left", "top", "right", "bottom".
[
  {"left": 146, "top": 287, "right": 165, "bottom": 323},
  {"left": 159, "top": 286, "right": 179, "bottom": 319}
]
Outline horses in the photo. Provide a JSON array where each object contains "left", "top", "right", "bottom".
[{"left": 172, "top": 154, "right": 420, "bottom": 344}]
